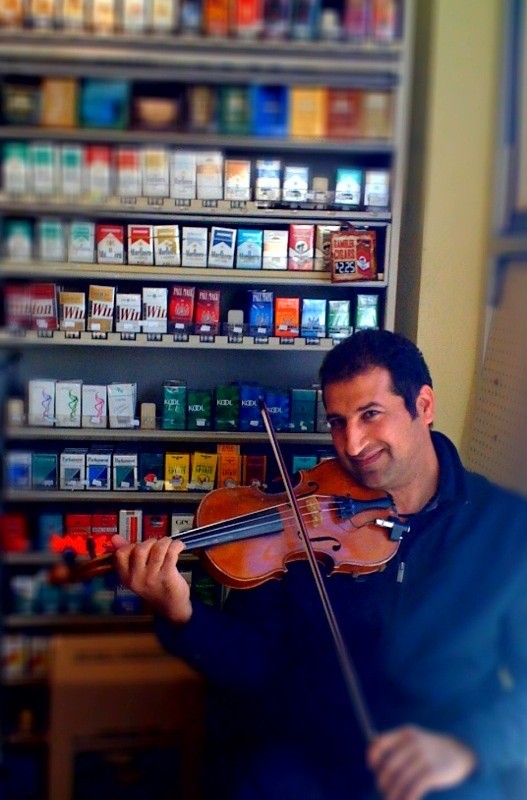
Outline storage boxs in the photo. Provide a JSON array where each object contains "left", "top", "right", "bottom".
[
  {"left": 28, "top": 377, "right": 137, "bottom": 428},
  {"left": 163, "top": 379, "right": 333, "bottom": 433},
  {"left": 5, "top": 446, "right": 339, "bottom": 491},
  {"left": 7, "top": 283, "right": 379, "bottom": 337},
  {"left": 116, "top": 582, "right": 143, "bottom": 615},
  {"left": 7, "top": 215, "right": 342, "bottom": 271},
  {"left": 3, "top": 142, "right": 390, "bottom": 205},
  {"left": 0, "top": 510, "right": 195, "bottom": 554}
]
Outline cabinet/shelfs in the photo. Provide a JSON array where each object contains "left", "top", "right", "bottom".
[{"left": 0, "top": 1, "right": 416, "bottom": 626}]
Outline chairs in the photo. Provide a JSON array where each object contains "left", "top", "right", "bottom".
[{"left": 48, "top": 632, "right": 202, "bottom": 800}]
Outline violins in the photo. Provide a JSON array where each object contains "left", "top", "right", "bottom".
[{"left": 45, "top": 457, "right": 402, "bottom": 589}]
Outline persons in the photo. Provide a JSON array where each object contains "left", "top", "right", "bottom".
[{"left": 113, "top": 328, "right": 527, "bottom": 800}]
